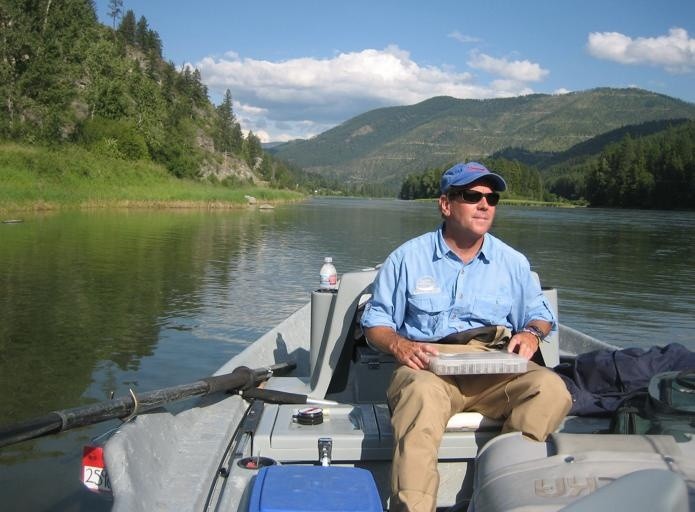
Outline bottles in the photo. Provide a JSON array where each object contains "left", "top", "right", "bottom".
[{"left": 320, "top": 257, "right": 337, "bottom": 291}]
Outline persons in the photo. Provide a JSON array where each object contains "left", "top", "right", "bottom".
[{"left": 357, "top": 161, "right": 574, "bottom": 511}]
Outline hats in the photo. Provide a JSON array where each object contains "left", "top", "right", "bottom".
[{"left": 439, "top": 160, "right": 509, "bottom": 197}]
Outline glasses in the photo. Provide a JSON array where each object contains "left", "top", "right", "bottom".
[{"left": 447, "top": 188, "right": 502, "bottom": 208}]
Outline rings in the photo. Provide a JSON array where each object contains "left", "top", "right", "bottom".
[{"left": 414, "top": 349, "right": 422, "bottom": 356}]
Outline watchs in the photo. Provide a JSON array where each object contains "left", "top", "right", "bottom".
[{"left": 524, "top": 325, "right": 546, "bottom": 342}]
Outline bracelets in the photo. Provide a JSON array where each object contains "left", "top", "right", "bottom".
[{"left": 517, "top": 327, "right": 543, "bottom": 346}]
